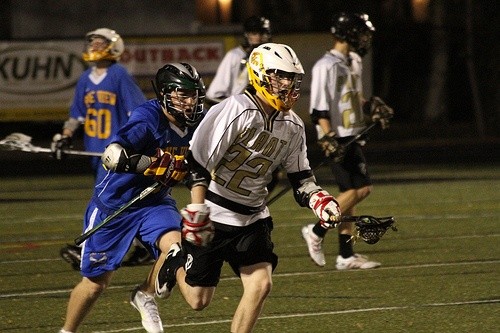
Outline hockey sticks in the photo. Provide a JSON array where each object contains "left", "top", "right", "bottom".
[
  {"left": 265, "top": 119, "right": 379, "bottom": 209},
  {"left": 0, "top": 133, "right": 102, "bottom": 157},
  {"left": 328, "top": 215, "right": 395, "bottom": 245},
  {"left": 74, "top": 181, "right": 162, "bottom": 246}
]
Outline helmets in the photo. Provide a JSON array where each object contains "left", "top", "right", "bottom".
[
  {"left": 331, "top": 10, "right": 375, "bottom": 58},
  {"left": 247, "top": 42, "right": 304, "bottom": 115},
  {"left": 153, "top": 62, "right": 208, "bottom": 127},
  {"left": 83, "top": 27, "right": 125, "bottom": 63},
  {"left": 244, "top": 15, "right": 272, "bottom": 50}
]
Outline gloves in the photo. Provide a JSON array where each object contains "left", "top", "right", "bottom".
[
  {"left": 179, "top": 203, "right": 215, "bottom": 247},
  {"left": 143, "top": 148, "right": 189, "bottom": 181},
  {"left": 316, "top": 130, "right": 344, "bottom": 163},
  {"left": 50, "top": 133, "right": 71, "bottom": 160},
  {"left": 309, "top": 190, "right": 342, "bottom": 229},
  {"left": 363, "top": 95, "right": 393, "bottom": 132}
]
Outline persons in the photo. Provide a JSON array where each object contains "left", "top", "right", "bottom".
[
  {"left": 302, "top": 11, "right": 394, "bottom": 269},
  {"left": 50, "top": 28, "right": 152, "bottom": 271},
  {"left": 60, "top": 61, "right": 206, "bottom": 333},
  {"left": 155, "top": 43, "right": 341, "bottom": 333},
  {"left": 205, "top": 16, "right": 271, "bottom": 99}
]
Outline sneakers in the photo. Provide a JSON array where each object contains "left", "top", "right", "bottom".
[
  {"left": 336, "top": 253, "right": 382, "bottom": 269},
  {"left": 130, "top": 285, "right": 164, "bottom": 333},
  {"left": 301, "top": 224, "right": 326, "bottom": 267},
  {"left": 155, "top": 242, "right": 181, "bottom": 299}
]
